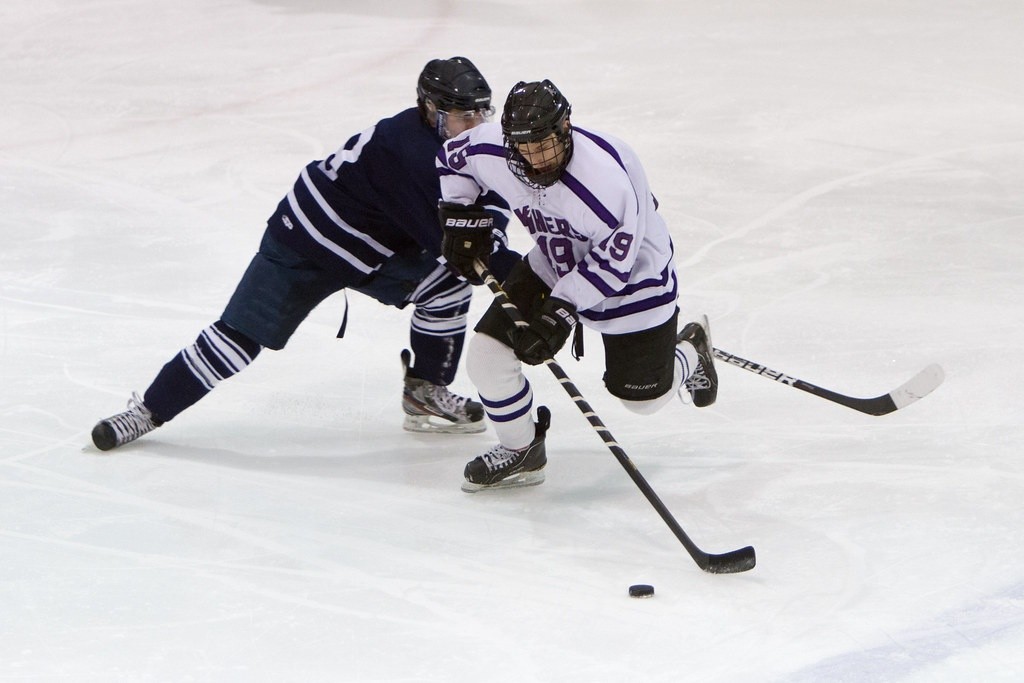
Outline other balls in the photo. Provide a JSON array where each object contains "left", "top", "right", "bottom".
[{"left": 628, "top": 584, "right": 655, "bottom": 598}]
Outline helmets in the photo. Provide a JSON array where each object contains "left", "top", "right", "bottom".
[
  {"left": 416, "top": 56, "right": 493, "bottom": 111},
  {"left": 501, "top": 80, "right": 574, "bottom": 191}
]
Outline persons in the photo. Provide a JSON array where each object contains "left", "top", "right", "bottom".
[
  {"left": 440, "top": 82, "right": 719, "bottom": 492},
  {"left": 88, "top": 59, "right": 513, "bottom": 454}
]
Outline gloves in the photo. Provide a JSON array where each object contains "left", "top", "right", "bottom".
[
  {"left": 512, "top": 297, "right": 578, "bottom": 366},
  {"left": 438, "top": 203, "right": 494, "bottom": 285}
]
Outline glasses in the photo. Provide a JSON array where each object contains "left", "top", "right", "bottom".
[{"left": 436, "top": 105, "right": 495, "bottom": 122}]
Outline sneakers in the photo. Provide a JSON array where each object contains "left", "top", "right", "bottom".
[
  {"left": 401, "top": 347, "right": 487, "bottom": 438},
  {"left": 460, "top": 408, "right": 552, "bottom": 494},
  {"left": 89, "top": 398, "right": 166, "bottom": 455},
  {"left": 677, "top": 313, "right": 718, "bottom": 410}
]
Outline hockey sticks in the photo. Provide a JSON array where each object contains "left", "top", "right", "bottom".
[
  {"left": 470, "top": 257, "right": 757, "bottom": 576},
  {"left": 707, "top": 343, "right": 948, "bottom": 420}
]
